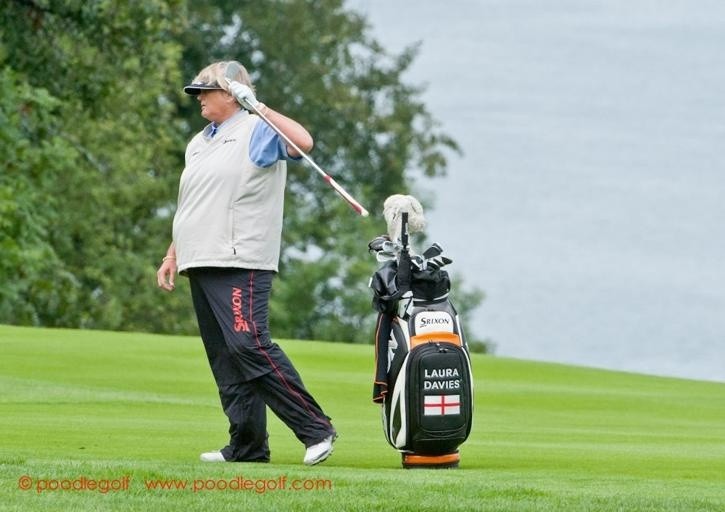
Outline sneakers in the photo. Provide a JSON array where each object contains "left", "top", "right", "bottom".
[
  {"left": 201, "top": 449, "right": 227, "bottom": 463},
  {"left": 305, "top": 430, "right": 338, "bottom": 467}
]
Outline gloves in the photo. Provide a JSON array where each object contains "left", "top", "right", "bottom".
[{"left": 226, "top": 83, "right": 263, "bottom": 114}]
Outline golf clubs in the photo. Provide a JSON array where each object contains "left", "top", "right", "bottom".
[
  {"left": 223, "top": 61, "right": 370, "bottom": 220},
  {"left": 368, "top": 233, "right": 453, "bottom": 271}
]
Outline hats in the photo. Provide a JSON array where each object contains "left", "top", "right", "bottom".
[{"left": 182, "top": 77, "right": 224, "bottom": 96}]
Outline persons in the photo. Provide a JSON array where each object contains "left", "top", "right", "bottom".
[{"left": 155, "top": 60, "right": 339, "bottom": 467}]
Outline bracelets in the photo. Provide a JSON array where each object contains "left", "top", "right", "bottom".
[
  {"left": 254, "top": 102, "right": 265, "bottom": 114},
  {"left": 161, "top": 255, "right": 177, "bottom": 261}
]
